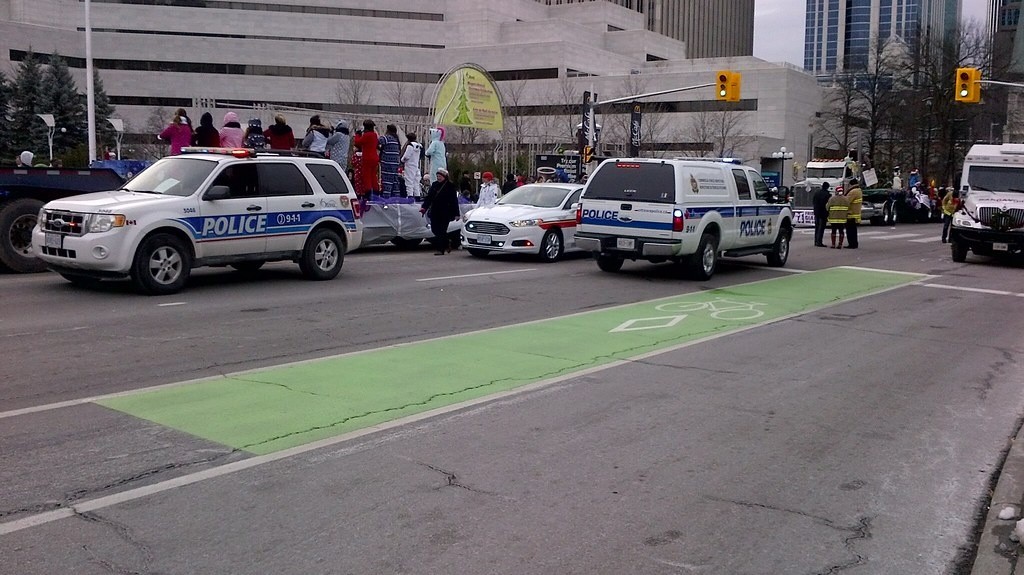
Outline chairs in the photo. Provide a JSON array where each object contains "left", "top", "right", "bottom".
[{"left": 236, "top": 164, "right": 259, "bottom": 197}]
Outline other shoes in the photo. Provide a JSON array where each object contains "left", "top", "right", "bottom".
[
  {"left": 434, "top": 251, "right": 444, "bottom": 255},
  {"left": 446, "top": 240, "right": 451, "bottom": 253},
  {"left": 949, "top": 240, "right": 952, "bottom": 243},
  {"left": 942, "top": 239, "right": 947, "bottom": 243},
  {"left": 844, "top": 245, "right": 851, "bottom": 248},
  {"left": 818, "top": 245, "right": 827, "bottom": 247}
]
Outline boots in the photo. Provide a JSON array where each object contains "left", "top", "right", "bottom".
[
  {"left": 836, "top": 234, "right": 844, "bottom": 249},
  {"left": 831, "top": 235, "right": 836, "bottom": 248}
]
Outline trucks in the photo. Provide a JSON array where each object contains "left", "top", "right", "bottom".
[
  {"left": 950, "top": 143, "right": 1024, "bottom": 267},
  {"left": 0, "top": 166, "right": 131, "bottom": 274}
]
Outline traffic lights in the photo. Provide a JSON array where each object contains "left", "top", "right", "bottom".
[
  {"left": 583, "top": 145, "right": 596, "bottom": 165},
  {"left": 716, "top": 71, "right": 731, "bottom": 100},
  {"left": 955, "top": 67, "right": 976, "bottom": 101}
]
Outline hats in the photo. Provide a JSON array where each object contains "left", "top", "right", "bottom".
[
  {"left": 310, "top": 115, "right": 320, "bottom": 123},
  {"left": 363, "top": 120, "right": 375, "bottom": 125},
  {"left": 836, "top": 186, "right": 843, "bottom": 191},
  {"left": 405, "top": 133, "right": 415, "bottom": 140},
  {"left": 823, "top": 182, "right": 829, "bottom": 188},
  {"left": 850, "top": 179, "right": 858, "bottom": 185},
  {"left": 436, "top": 168, "right": 448, "bottom": 176}
]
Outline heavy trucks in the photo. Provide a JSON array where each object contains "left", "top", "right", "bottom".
[{"left": 803, "top": 159, "right": 946, "bottom": 227}]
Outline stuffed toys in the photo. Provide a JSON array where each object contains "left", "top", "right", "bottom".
[{"left": 425, "top": 127, "right": 447, "bottom": 186}]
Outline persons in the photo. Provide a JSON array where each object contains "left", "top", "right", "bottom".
[
  {"left": 103, "top": 148, "right": 111, "bottom": 160},
  {"left": 908, "top": 168, "right": 922, "bottom": 189},
  {"left": 191, "top": 112, "right": 220, "bottom": 146},
  {"left": 301, "top": 115, "right": 330, "bottom": 154},
  {"left": 11, "top": 151, "right": 34, "bottom": 167},
  {"left": 843, "top": 178, "right": 862, "bottom": 249},
  {"left": 157, "top": 108, "right": 193, "bottom": 155},
  {"left": 788, "top": 186, "right": 794, "bottom": 197},
  {"left": 942, "top": 190, "right": 959, "bottom": 243},
  {"left": 263, "top": 114, "right": 296, "bottom": 150},
  {"left": 826, "top": 186, "right": 850, "bottom": 250},
  {"left": 327, "top": 119, "right": 350, "bottom": 172},
  {"left": 812, "top": 182, "right": 830, "bottom": 247},
  {"left": 456, "top": 171, "right": 526, "bottom": 207},
  {"left": 347, "top": 119, "right": 423, "bottom": 203},
  {"left": 418, "top": 168, "right": 460, "bottom": 256},
  {"left": 219, "top": 112, "right": 245, "bottom": 147},
  {"left": 243, "top": 118, "right": 266, "bottom": 149}
]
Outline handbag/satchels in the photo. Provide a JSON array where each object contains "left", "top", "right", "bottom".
[{"left": 427, "top": 210, "right": 431, "bottom": 218}]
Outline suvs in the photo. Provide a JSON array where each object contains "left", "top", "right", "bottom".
[
  {"left": 32, "top": 146, "right": 364, "bottom": 297},
  {"left": 573, "top": 157, "right": 794, "bottom": 281}
]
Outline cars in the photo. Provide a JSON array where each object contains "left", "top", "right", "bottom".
[{"left": 459, "top": 183, "right": 594, "bottom": 263}]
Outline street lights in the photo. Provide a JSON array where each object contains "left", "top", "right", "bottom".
[{"left": 771, "top": 146, "right": 795, "bottom": 185}]
